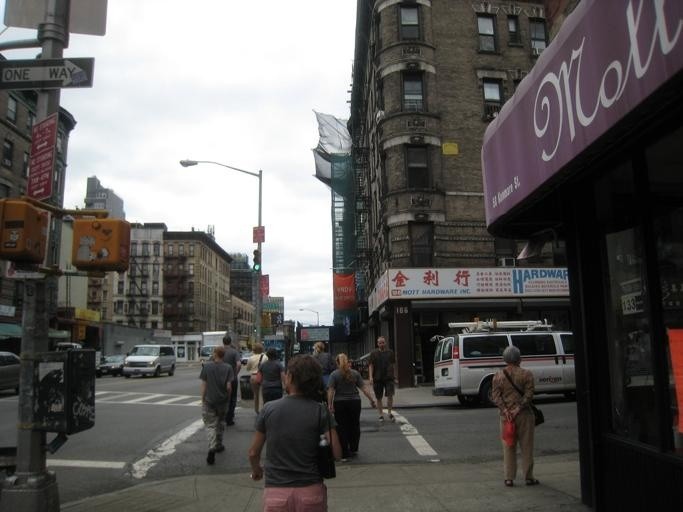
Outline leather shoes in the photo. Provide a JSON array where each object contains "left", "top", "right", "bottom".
[
  {"left": 216, "top": 444, "right": 224, "bottom": 452},
  {"left": 206, "top": 450, "right": 215, "bottom": 464},
  {"left": 227, "top": 420, "right": 234, "bottom": 426}
]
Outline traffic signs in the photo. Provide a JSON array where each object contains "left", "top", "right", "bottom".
[{"left": 0, "top": 57, "right": 92, "bottom": 89}]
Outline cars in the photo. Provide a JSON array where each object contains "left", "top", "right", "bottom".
[
  {"left": 94, "top": 352, "right": 123, "bottom": 378},
  {"left": 0, "top": 352, "right": 22, "bottom": 395}
]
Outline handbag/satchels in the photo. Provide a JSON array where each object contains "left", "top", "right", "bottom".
[
  {"left": 318, "top": 406, "right": 336, "bottom": 480},
  {"left": 533, "top": 407, "right": 543, "bottom": 426},
  {"left": 255, "top": 370, "right": 263, "bottom": 385},
  {"left": 502, "top": 415, "right": 515, "bottom": 446}
]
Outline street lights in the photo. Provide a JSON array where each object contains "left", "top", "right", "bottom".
[
  {"left": 178, "top": 158, "right": 263, "bottom": 336},
  {"left": 299, "top": 308, "right": 319, "bottom": 326}
]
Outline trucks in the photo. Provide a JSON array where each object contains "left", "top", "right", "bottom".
[{"left": 199, "top": 332, "right": 231, "bottom": 371}]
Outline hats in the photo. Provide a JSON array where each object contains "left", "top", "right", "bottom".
[{"left": 503, "top": 345, "right": 520, "bottom": 363}]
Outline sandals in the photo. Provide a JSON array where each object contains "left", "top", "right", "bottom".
[
  {"left": 504, "top": 480, "right": 512, "bottom": 487},
  {"left": 525, "top": 479, "right": 539, "bottom": 486}
]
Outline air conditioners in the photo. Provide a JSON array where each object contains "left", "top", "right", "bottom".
[{"left": 533, "top": 47, "right": 545, "bottom": 56}]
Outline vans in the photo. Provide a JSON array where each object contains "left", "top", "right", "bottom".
[
  {"left": 122, "top": 344, "right": 175, "bottom": 378},
  {"left": 431, "top": 320, "right": 574, "bottom": 405}
]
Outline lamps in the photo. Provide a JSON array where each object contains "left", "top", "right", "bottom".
[{"left": 516, "top": 228, "right": 569, "bottom": 265}]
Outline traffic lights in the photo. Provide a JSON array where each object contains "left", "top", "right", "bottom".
[{"left": 252, "top": 250, "right": 261, "bottom": 272}]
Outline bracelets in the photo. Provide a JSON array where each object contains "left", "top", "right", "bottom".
[{"left": 372, "top": 400, "right": 375, "bottom": 404}]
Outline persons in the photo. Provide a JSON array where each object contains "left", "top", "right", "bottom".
[
  {"left": 246, "top": 355, "right": 344, "bottom": 511},
  {"left": 247, "top": 343, "right": 268, "bottom": 415},
  {"left": 489, "top": 344, "right": 541, "bottom": 489},
  {"left": 258, "top": 348, "right": 286, "bottom": 403},
  {"left": 368, "top": 335, "right": 399, "bottom": 423},
  {"left": 210, "top": 335, "right": 242, "bottom": 426},
  {"left": 312, "top": 342, "right": 336, "bottom": 403},
  {"left": 199, "top": 346, "right": 235, "bottom": 463},
  {"left": 326, "top": 352, "right": 375, "bottom": 463}
]
